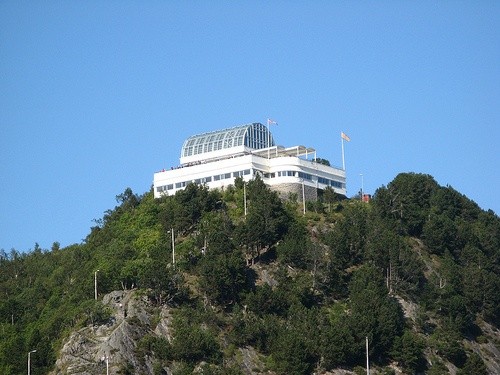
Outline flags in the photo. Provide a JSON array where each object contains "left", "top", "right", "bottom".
[
  {"left": 268, "top": 119, "right": 277, "bottom": 125},
  {"left": 342, "top": 133, "right": 350, "bottom": 142}
]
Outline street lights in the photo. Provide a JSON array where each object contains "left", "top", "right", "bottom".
[
  {"left": 364, "top": 333, "right": 369, "bottom": 375},
  {"left": 94, "top": 269, "right": 100, "bottom": 299},
  {"left": 166, "top": 226, "right": 176, "bottom": 271},
  {"left": 27, "top": 349, "right": 37, "bottom": 375},
  {"left": 242, "top": 177, "right": 248, "bottom": 216},
  {"left": 358, "top": 172, "right": 365, "bottom": 199},
  {"left": 297, "top": 178, "right": 307, "bottom": 215}
]
